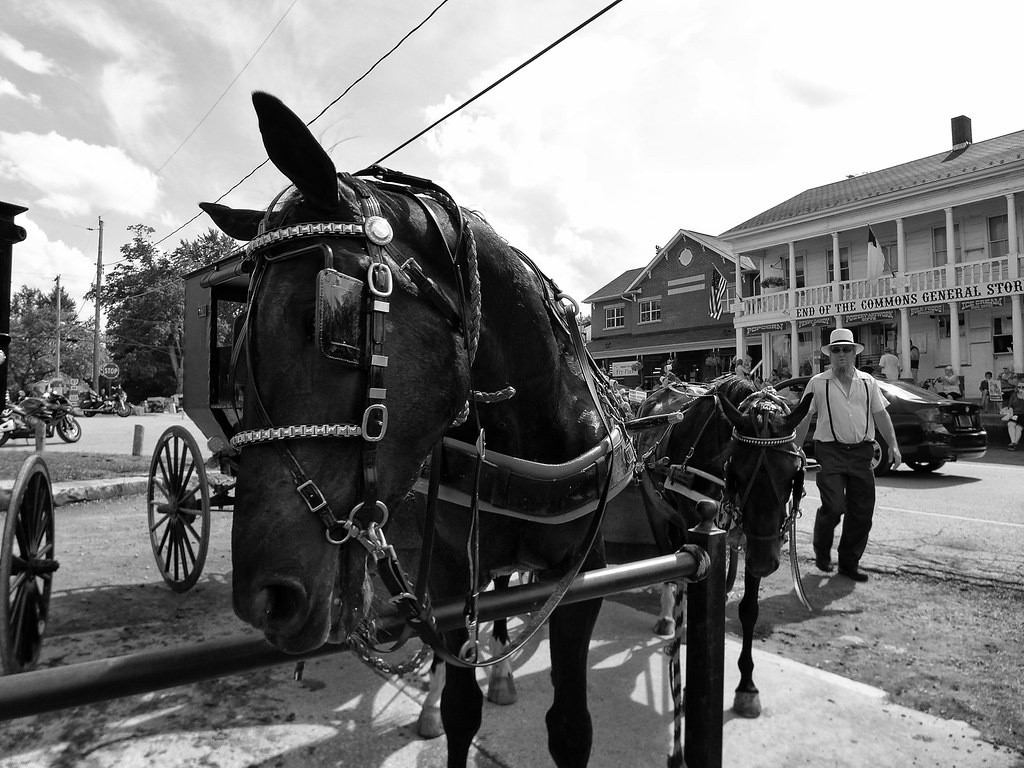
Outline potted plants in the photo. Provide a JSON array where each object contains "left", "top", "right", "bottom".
[{"left": 758, "top": 276, "right": 787, "bottom": 290}]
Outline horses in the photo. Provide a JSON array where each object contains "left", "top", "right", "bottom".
[
  {"left": 198, "top": 91, "right": 615, "bottom": 768},
  {"left": 633, "top": 374, "right": 814, "bottom": 718}
]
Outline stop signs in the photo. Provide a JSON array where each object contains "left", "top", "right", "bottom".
[{"left": 103, "top": 363, "right": 119, "bottom": 379}]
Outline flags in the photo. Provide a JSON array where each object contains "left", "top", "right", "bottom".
[
  {"left": 706, "top": 265, "right": 728, "bottom": 321},
  {"left": 867, "top": 227, "right": 885, "bottom": 286}
]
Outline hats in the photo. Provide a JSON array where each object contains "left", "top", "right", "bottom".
[
  {"left": 945, "top": 366, "right": 952, "bottom": 370},
  {"left": 821, "top": 329, "right": 864, "bottom": 356},
  {"left": 884, "top": 347, "right": 891, "bottom": 351}
]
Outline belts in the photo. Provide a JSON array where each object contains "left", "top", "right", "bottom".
[{"left": 828, "top": 441, "right": 868, "bottom": 449}]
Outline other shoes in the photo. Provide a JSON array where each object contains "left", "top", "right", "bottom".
[
  {"left": 839, "top": 566, "right": 868, "bottom": 580},
  {"left": 813, "top": 548, "right": 832, "bottom": 572},
  {"left": 1008, "top": 443, "right": 1019, "bottom": 451}
]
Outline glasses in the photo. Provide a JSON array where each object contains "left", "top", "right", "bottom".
[
  {"left": 1017, "top": 386, "right": 1024, "bottom": 388},
  {"left": 830, "top": 346, "right": 855, "bottom": 353},
  {"left": 1003, "top": 368, "right": 1007, "bottom": 369}
]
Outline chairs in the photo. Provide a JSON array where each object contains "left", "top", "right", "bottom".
[{"left": 957, "top": 376, "right": 966, "bottom": 401}]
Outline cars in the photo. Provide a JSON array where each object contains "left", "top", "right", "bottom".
[{"left": 767, "top": 374, "right": 988, "bottom": 475}]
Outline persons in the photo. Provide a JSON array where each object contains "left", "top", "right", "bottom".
[
  {"left": 937, "top": 366, "right": 962, "bottom": 400},
  {"left": 910, "top": 338, "right": 920, "bottom": 385},
  {"left": 792, "top": 329, "right": 901, "bottom": 582},
  {"left": 17, "top": 385, "right": 108, "bottom": 437},
  {"left": 859, "top": 347, "right": 903, "bottom": 381},
  {"left": 735, "top": 359, "right": 783, "bottom": 390},
  {"left": 979, "top": 364, "right": 1024, "bottom": 451}
]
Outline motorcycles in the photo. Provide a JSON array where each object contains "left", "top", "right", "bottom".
[
  {"left": 81, "top": 384, "right": 131, "bottom": 418},
  {"left": 0, "top": 384, "right": 82, "bottom": 445}
]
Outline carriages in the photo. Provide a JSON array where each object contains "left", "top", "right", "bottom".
[{"left": 0, "top": 92, "right": 814, "bottom": 768}]
